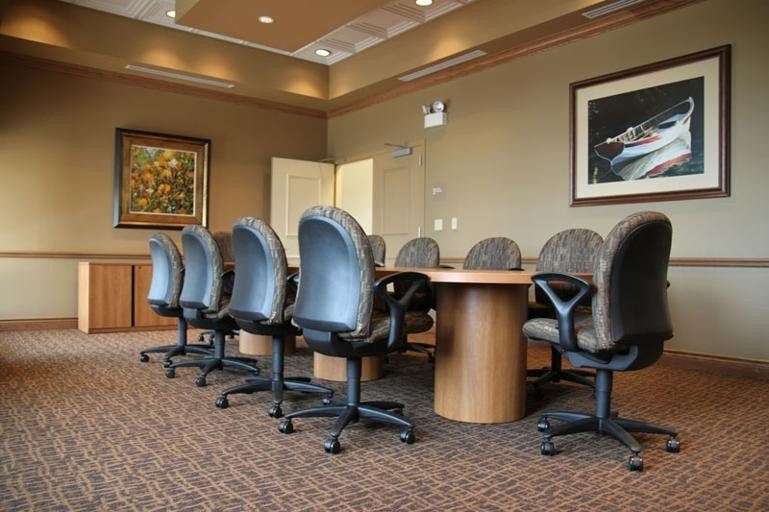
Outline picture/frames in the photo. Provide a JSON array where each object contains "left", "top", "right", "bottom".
[
  {"left": 111, "top": 128, "right": 211, "bottom": 231},
  {"left": 566, "top": 43, "right": 733, "bottom": 207}
]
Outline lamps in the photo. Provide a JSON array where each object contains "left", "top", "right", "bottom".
[{"left": 422, "top": 100, "right": 448, "bottom": 129}]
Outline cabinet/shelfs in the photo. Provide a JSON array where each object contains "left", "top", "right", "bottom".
[{"left": 77, "top": 261, "right": 195, "bottom": 335}]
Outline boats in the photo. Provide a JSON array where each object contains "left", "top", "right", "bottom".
[
  {"left": 618, "top": 128, "right": 692, "bottom": 181},
  {"left": 592, "top": 96, "right": 695, "bottom": 168}
]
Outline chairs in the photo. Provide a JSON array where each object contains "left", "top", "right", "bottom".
[
  {"left": 139, "top": 234, "right": 214, "bottom": 367},
  {"left": 463, "top": 237, "right": 522, "bottom": 272},
  {"left": 277, "top": 206, "right": 416, "bottom": 455},
  {"left": 366, "top": 234, "right": 387, "bottom": 268},
  {"left": 394, "top": 238, "right": 435, "bottom": 361},
  {"left": 198, "top": 231, "right": 240, "bottom": 344},
  {"left": 215, "top": 217, "right": 333, "bottom": 418},
  {"left": 522, "top": 212, "right": 682, "bottom": 471},
  {"left": 166, "top": 223, "right": 261, "bottom": 388},
  {"left": 527, "top": 228, "right": 596, "bottom": 391}
]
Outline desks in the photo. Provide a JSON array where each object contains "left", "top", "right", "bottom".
[{"left": 221, "top": 260, "right": 593, "bottom": 425}]
